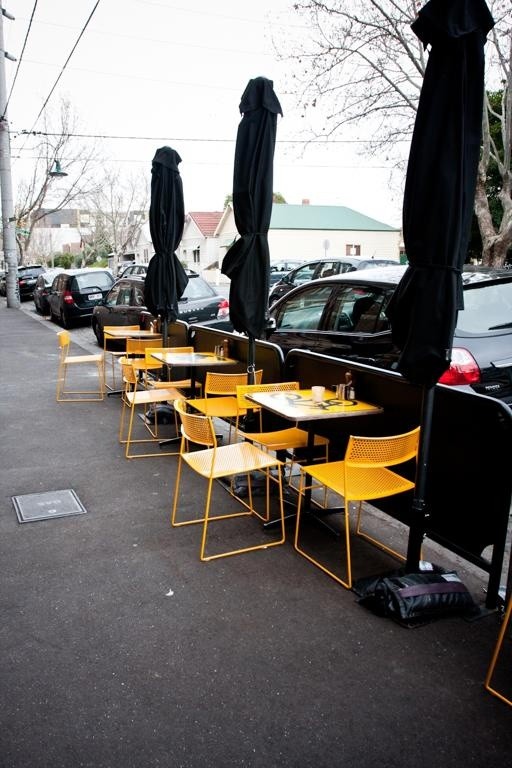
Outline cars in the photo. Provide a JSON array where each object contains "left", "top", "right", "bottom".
[
  {"left": 233, "top": 264, "right": 511, "bottom": 496},
  {"left": 1, "top": 249, "right": 402, "bottom": 351}
]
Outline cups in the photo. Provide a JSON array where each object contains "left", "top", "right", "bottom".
[{"left": 312, "top": 386, "right": 325, "bottom": 403}]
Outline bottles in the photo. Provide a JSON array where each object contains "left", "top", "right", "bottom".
[
  {"left": 214, "top": 339, "right": 229, "bottom": 357},
  {"left": 333, "top": 372, "right": 356, "bottom": 400},
  {"left": 150, "top": 314, "right": 162, "bottom": 334}
]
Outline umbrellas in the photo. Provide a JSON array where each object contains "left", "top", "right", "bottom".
[
  {"left": 144, "top": 146, "right": 191, "bottom": 404},
  {"left": 219, "top": 75, "right": 284, "bottom": 441},
  {"left": 380, "top": 0, "right": 499, "bottom": 572}
]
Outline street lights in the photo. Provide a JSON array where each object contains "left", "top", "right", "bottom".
[{"left": 0, "top": 124, "right": 69, "bottom": 312}]
